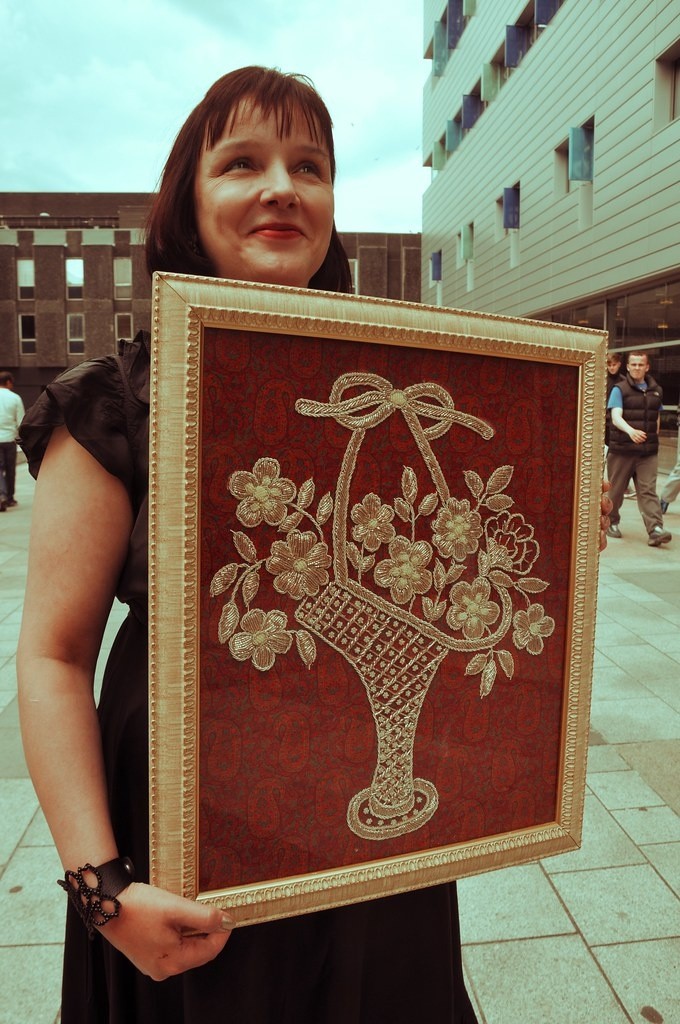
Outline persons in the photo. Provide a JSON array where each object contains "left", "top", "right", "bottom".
[
  {"left": 0, "top": 372, "right": 26, "bottom": 512},
  {"left": 660, "top": 403, "right": 680, "bottom": 514},
  {"left": 18, "top": 67, "right": 613, "bottom": 1024},
  {"left": 607, "top": 352, "right": 672, "bottom": 546}
]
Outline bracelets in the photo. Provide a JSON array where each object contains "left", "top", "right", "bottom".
[{"left": 57, "top": 856, "right": 135, "bottom": 937}]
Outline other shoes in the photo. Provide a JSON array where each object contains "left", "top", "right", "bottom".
[
  {"left": 606, "top": 524, "right": 621, "bottom": 538},
  {"left": 623, "top": 487, "right": 637, "bottom": 498},
  {"left": 0, "top": 495, "right": 17, "bottom": 512},
  {"left": 661, "top": 499, "right": 669, "bottom": 514},
  {"left": 648, "top": 526, "right": 672, "bottom": 546}
]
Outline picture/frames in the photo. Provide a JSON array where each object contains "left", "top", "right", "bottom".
[{"left": 151, "top": 273, "right": 606, "bottom": 938}]
